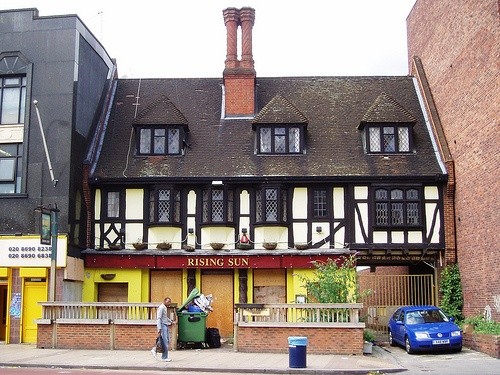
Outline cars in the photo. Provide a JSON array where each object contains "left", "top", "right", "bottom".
[{"left": 387, "top": 305, "right": 463, "bottom": 355}]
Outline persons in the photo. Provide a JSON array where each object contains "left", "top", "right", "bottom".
[{"left": 151, "top": 297, "right": 176, "bottom": 362}]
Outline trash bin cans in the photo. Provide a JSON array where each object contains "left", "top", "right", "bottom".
[
  {"left": 176, "top": 288, "right": 208, "bottom": 349},
  {"left": 288, "top": 336, "right": 307, "bottom": 368}
]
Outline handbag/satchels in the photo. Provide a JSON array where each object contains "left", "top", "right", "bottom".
[{"left": 156, "top": 336, "right": 165, "bottom": 353}]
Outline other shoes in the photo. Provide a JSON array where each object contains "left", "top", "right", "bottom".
[
  {"left": 150, "top": 349, "right": 158, "bottom": 359},
  {"left": 161, "top": 358, "right": 172, "bottom": 362}
]
